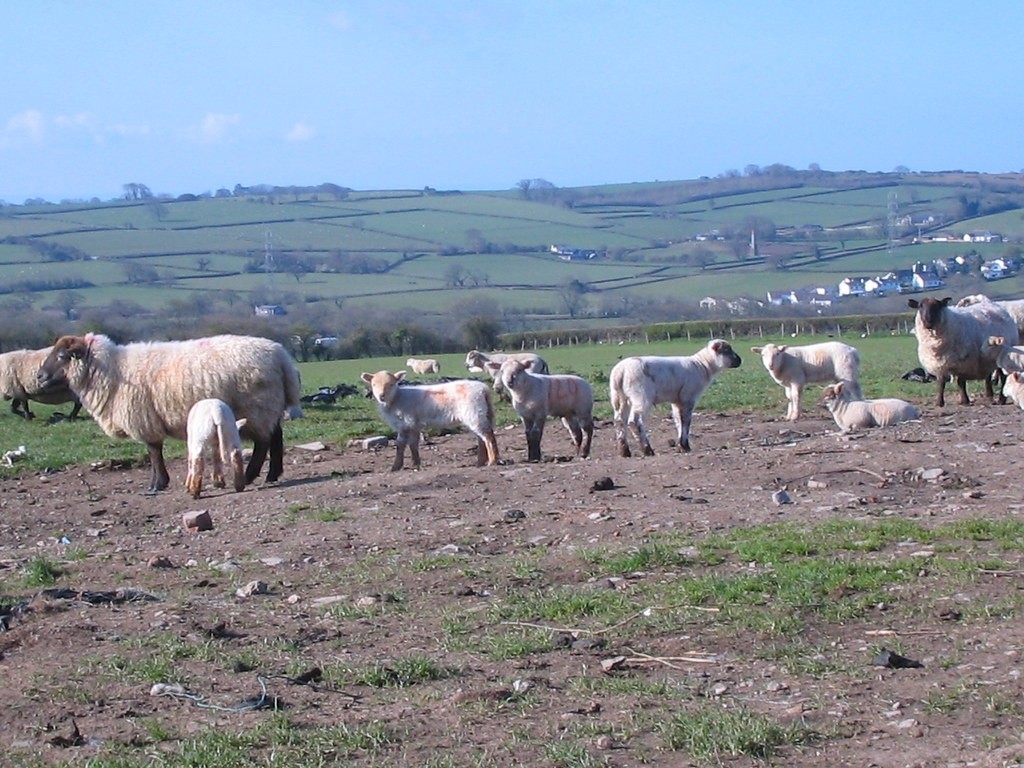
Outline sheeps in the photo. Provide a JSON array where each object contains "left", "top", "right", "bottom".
[
  {"left": 464, "top": 350, "right": 550, "bottom": 402},
  {"left": 749, "top": 342, "right": 862, "bottom": 422},
  {"left": 1, "top": 332, "right": 303, "bottom": 499},
  {"left": 908, "top": 292, "right": 1024, "bottom": 411},
  {"left": 359, "top": 370, "right": 500, "bottom": 472},
  {"left": 818, "top": 380, "right": 917, "bottom": 434},
  {"left": 485, "top": 359, "right": 596, "bottom": 463},
  {"left": 406, "top": 358, "right": 441, "bottom": 376},
  {"left": 609, "top": 338, "right": 744, "bottom": 457}
]
